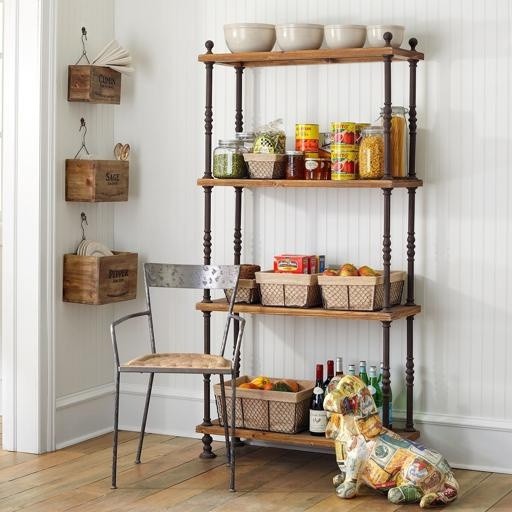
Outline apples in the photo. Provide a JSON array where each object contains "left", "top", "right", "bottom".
[{"left": 321, "top": 263, "right": 383, "bottom": 276}]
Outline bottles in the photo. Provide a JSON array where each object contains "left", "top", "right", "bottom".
[
  {"left": 308, "top": 357, "right": 393, "bottom": 437},
  {"left": 358, "top": 104, "right": 411, "bottom": 179},
  {"left": 213, "top": 132, "right": 256, "bottom": 179}
]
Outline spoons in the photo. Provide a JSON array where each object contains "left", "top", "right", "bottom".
[{"left": 114, "top": 142, "right": 130, "bottom": 161}]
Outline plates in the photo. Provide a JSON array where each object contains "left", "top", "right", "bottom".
[{"left": 76, "top": 240, "right": 114, "bottom": 257}]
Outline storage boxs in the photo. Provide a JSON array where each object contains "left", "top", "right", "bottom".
[
  {"left": 66, "top": 159, "right": 129, "bottom": 203},
  {"left": 67, "top": 65, "right": 122, "bottom": 104},
  {"left": 63, "top": 251, "right": 138, "bottom": 305}
]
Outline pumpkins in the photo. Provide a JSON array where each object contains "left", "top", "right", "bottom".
[{"left": 238, "top": 377, "right": 299, "bottom": 393}]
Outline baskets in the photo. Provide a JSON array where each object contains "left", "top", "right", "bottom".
[
  {"left": 225, "top": 264, "right": 261, "bottom": 305},
  {"left": 244, "top": 154, "right": 285, "bottom": 180},
  {"left": 255, "top": 272, "right": 319, "bottom": 307},
  {"left": 214, "top": 375, "right": 316, "bottom": 434},
  {"left": 319, "top": 270, "right": 405, "bottom": 312}
]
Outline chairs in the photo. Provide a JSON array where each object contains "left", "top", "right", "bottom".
[{"left": 110, "top": 262, "right": 246, "bottom": 491}]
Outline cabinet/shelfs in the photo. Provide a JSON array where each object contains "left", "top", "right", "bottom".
[{"left": 197, "top": 33, "right": 425, "bottom": 460}]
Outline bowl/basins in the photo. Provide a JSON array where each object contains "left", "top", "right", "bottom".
[
  {"left": 366, "top": 24, "right": 405, "bottom": 49},
  {"left": 223, "top": 22, "right": 276, "bottom": 54},
  {"left": 274, "top": 22, "right": 324, "bottom": 51},
  {"left": 323, "top": 24, "right": 367, "bottom": 50}
]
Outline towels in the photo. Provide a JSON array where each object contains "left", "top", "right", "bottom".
[{"left": 91, "top": 40, "right": 135, "bottom": 76}]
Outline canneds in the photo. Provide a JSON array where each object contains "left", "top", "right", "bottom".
[
  {"left": 213, "top": 140, "right": 249, "bottom": 179},
  {"left": 295, "top": 123, "right": 319, "bottom": 140},
  {"left": 331, "top": 151, "right": 358, "bottom": 180},
  {"left": 295, "top": 139, "right": 319, "bottom": 153},
  {"left": 358, "top": 129, "right": 385, "bottom": 179},
  {"left": 304, "top": 158, "right": 330, "bottom": 179},
  {"left": 235, "top": 132, "right": 256, "bottom": 154},
  {"left": 356, "top": 124, "right": 372, "bottom": 151},
  {"left": 304, "top": 153, "right": 319, "bottom": 158},
  {"left": 285, "top": 151, "right": 304, "bottom": 179},
  {"left": 330, "top": 122, "right": 356, "bottom": 151}
]
